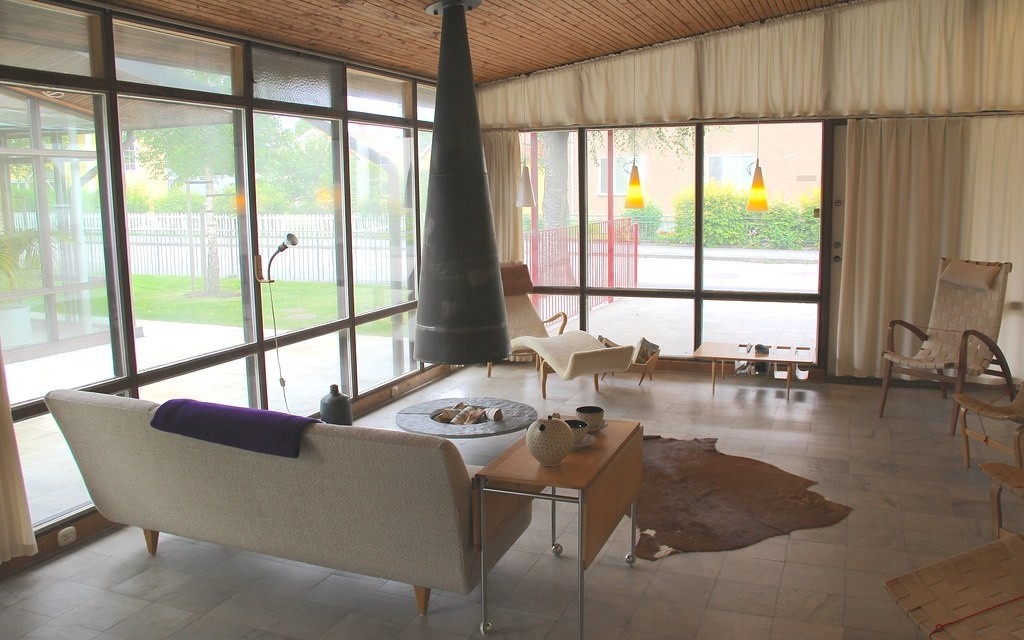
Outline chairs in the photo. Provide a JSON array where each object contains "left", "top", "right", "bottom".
[
  {"left": 883, "top": 382, "right": 1024, "bottom": 640},
  {"left": 485, "top": 260, "right": 635, "bottom": 399},
  {"left": 879, "top": 255, "right": 1023, "bottom": 434}
]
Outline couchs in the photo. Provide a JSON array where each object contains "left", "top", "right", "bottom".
[{"left": 46, "top": 391, "right": 534, "bottom": 615}]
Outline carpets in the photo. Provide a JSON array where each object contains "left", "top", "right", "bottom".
[{"left": 614, "top": 431, "right": 855, "bottom": 560}]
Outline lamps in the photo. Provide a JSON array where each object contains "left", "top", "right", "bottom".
[
  {"left": 625, "top": 47, "right": 646, "bottom": 208},
  {"left": 516, "top": 40, "right": 536, "bottom": 210},
  {"left": 748, "top": 22, "right": 769, "bottom": 214},
  {"left": 266, "top": 233, "right": 299, "bottom": 384}
]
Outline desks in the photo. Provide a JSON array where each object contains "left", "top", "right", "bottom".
[
  {"left": 470, "top": 412, "right": 649, "bottom": 639},
  {"left": 695, "top": 343, "right": 814, "bottom": 400}
]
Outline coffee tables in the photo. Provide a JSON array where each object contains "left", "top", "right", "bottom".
[{"left": 395, "top": 397, "right": 539, "bottom": 440}]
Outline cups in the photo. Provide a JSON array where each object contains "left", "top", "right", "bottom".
[
  {"left": 575, "top": 406, "right": 604, "bottom": 430},
  {"left": 563, "top": 420, "right": 589, "bottom": 443}
]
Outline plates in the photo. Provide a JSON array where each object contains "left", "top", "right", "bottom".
[
  {"left": 570, "top": 434, "right": 596, "bottom": 450},
  {"left": 587, "top": 419, "right": 608, "bottom": 433}
]
